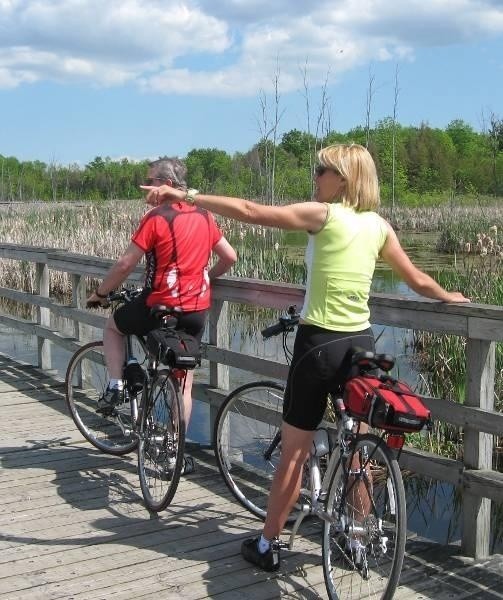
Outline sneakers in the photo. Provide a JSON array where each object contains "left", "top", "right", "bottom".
[
  {"left": 337, "top": 532, "right": 366, "bottom": 573},
  {"left": 161, "top": 449, "right": 195, "bottom": 481},
  {"left": 238, "top": 536, "right": 282, "bottom": 572},
  {"left": 96, "top": 381, "right": 126, "bottom": 412}
]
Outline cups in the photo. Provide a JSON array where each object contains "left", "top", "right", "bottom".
[{"left": 126, "top": 357, "right": 144, "bottom": 391}]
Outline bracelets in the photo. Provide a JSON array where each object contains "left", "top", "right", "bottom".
[
  {"left": 185, "top": 187, "right": 198, "bottom": 207},
  {"left": 94, "top": 287, "right": 108, "bottom": 299}
]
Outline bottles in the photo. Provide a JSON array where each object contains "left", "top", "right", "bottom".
[
  {"left": 309, "top": 420, "right": 330, "bottom": 458},
  {"left": 335, "top": 396, "right": 370, "bottom": 476}
]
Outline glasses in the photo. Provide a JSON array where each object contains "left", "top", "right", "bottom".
[
  {"left": 141, "top": 176, "right": 168, "bottom": 186},
  {"left": 312, "top": 165, "right": 330, "bottom": 177}
]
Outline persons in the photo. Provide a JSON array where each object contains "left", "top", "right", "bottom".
[
  {"left": 84, "top": 157, "right": 237, "bottom": 478},
  {"left": 139, "top": 138, "right": 471, "bottom": 571}
]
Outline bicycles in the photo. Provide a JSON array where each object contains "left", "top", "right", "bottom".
[
  {"left": 64, "top": 285, "right": 202, "bottom": 512},
  {"left": 212, "top": 304, "right": 435, "bottom": 600}
]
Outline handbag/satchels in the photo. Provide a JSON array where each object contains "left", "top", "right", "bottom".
[{"left": 342, "top": 373, "right": 435, "bottom": 434}]
